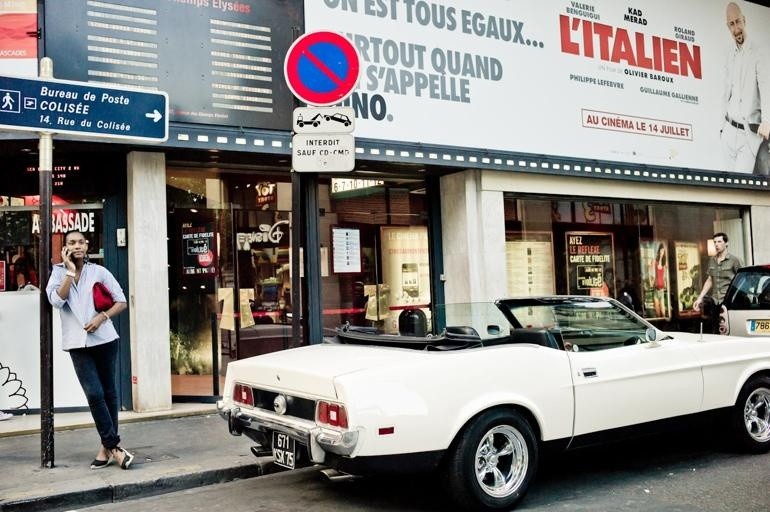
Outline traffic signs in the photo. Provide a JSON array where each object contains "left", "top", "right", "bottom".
[{"left": 0, "top": 76, "right": 169, "bottom": 146}]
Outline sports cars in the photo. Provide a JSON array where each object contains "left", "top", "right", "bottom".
[{"left": 214, "top": 295, "right": 770, "bottom": 509}]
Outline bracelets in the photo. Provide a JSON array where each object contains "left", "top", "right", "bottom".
[
  {"left": 66, "top": 271, "right": 78, "bottom": 278},
  {"left": 103, "top": 312, "right": 110, "bottom": 319}
]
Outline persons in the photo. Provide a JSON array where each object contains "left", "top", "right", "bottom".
[
  {"left": 722, "top": 2, "right": 770, "bottom": 174},
  {"left": 45, "top": 230, "right": 134, "bottom": 469},
  {"left": 649, "top": 245, "right": 667, "bottom": 317},
  {"left": 692, "top": 233, "right": 742, "bottom": 335}
]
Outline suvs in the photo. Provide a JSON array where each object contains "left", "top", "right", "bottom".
[{"left": 720, "top": 264, "right": 770, "bottom": 336}]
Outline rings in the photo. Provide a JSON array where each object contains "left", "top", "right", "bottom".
[{"left": 90, "top": 331, "right": 93, "bottom": 333}]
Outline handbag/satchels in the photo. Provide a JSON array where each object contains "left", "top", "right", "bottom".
[{"left": 92, "top": 282, "right": 114, "bottom": 313}]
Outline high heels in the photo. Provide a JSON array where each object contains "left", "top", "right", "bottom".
[
  {"left": 121, "top": 448, "right": 133, "bottom": 469},
  {"left": 90, "top": 454, "right": 114, "bottom": 469}
]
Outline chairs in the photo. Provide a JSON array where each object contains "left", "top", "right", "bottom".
[{"left": 435, "top": 324, "right": 562, "bottom": 349}]
